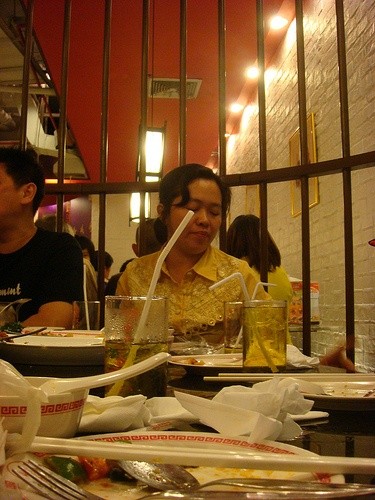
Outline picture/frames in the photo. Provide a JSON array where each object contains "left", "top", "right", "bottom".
[
  {"left": 288, "top": 112, "right": 319, "bottom": 218},
  {"left": 243, "top": 184, "right": 261, "bottom": 217}
]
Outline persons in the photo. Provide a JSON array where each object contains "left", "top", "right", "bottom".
[
  {"left": 224, "top": 213, "right": 295, "bottom": 304},
  {"left": 132, "top": 218, "right": 165, "bottom": 259},
  {"left": 105, "top": 258, "right": 137, "bottom": 297},
  {"left": 95, "top": 249, "right": 113, "bottom": 289},
  {"left": 0, "top": 145, "right": 81, "bottom": 333},
  {"left": 74, "top": 234, "right": 97, "bottom": 272},
  {"left": 34, "top": 215, "right": 100, "bottom": 306},
  {"left": 114, "top": 163, "right": 364, "bottom": 374}
]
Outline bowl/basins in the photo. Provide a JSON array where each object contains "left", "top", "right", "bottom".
[{"left": 0, "top": 376, "right": 90, "bottom": 438}]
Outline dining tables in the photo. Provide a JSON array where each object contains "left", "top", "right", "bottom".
[{"left": 165, "top": 364, "right": 375, "bottom": 500}]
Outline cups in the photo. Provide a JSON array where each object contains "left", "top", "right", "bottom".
[
  {"left": 242, "top": 299, "right": 288, "bottom": 374},
  {"left": 102, "top": 294, "right": 168, "bottom": 396},
  {"left": 223, "top": 301, "right": 243, "bottom": 352},
  {"left": 72, "top": 300, "right": 100, "bottom": 331}
]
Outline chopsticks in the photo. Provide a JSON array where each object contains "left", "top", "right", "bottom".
[
  {"left": 203, "top": 373, "right": 375, "bottom": 382},
  {"left": 29, "top": 435, "right": 375, "bottom": 475}
]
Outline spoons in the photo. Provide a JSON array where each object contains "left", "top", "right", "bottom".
[{"left": 37, "top": 351, "right": 171, "bottom": 395}]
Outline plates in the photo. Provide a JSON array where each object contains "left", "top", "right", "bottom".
[
  {"left": 22, "top": 326, "right": 103, "bottom": 337},
  {"left": 298, "top": 381, "right": 375, "bottom": 411},
  {"left": 0, "top": 335, "right": 104, "bottom": 366},
  {"left": 0, "top": 430, "right": 345, "bottom": 499},
  {"left": 166, "top": 354, "right": 242, "bottom": 374}
]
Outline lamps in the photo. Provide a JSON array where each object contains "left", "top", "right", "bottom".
[{"left": 128, "top": 0, "right": 167, "bottom": 228}]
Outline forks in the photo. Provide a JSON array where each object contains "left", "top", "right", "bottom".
[
  {"left": 118, "top": 460, "right": 375, "bottom": 493},
  {"left": 12, "top": 459, "right": 375, "bottom": 500}
]
